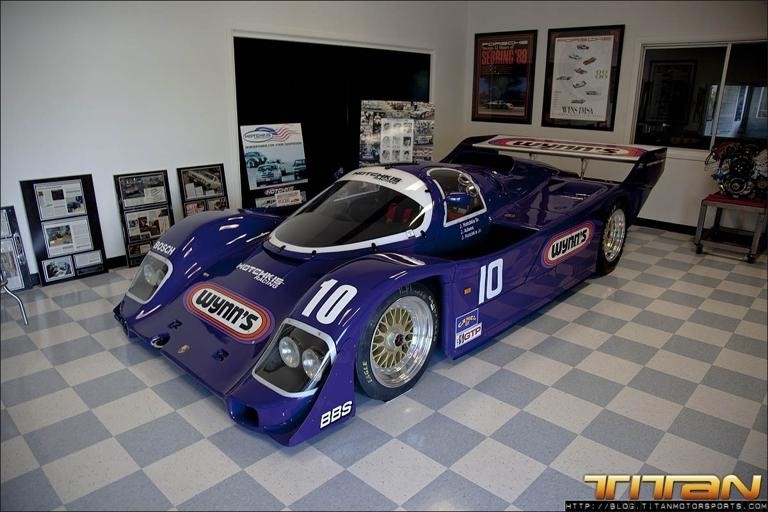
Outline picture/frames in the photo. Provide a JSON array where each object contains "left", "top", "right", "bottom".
[
  {"left": 470, "top": 22, "right": 627, "bottom": 133},
  {"left": 28, "top": 179, "right": 108, "bottom": 285},
  {"left": 642, "top": 60, "right": 699, "bottom": 126},
  {"left": 116, "top": 165, "right": 227, "bottom": 269}
]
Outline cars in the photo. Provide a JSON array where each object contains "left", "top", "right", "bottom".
[
  {"left": 46, "top": 228, "right": 65, "bottom": 246},
  {"left": 56, "top": 261, "right": 71, "bottom": 269},
  {"left": 66, "top": 201, "right": 80, "bottom": 210}
]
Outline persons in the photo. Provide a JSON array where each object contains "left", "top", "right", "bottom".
[{"left": 358, "top": 99, "right": 436, "bottom": 167}]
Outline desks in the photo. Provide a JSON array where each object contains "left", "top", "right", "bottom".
[{"left": 692, "top": 193, "right": 766, "bottom": 264}]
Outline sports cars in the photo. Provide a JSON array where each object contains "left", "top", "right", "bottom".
[
  {"left": 487, "top": 99, "right": 513, "bottom": 111},
  {"left": 555, "top": 41, "right": 600, "bottom": 103},
  {"left": 115, "top": 169, "right": 227, "bottom": 258},
  {"left": 360, "top": 100, "right": 434, "bottom": 165},
  {"left": 110, "top": 132, "right": 668, "bottom": 448},
  {"left": 242, "top": 150, "right": 307, "bottom": 187}
]
